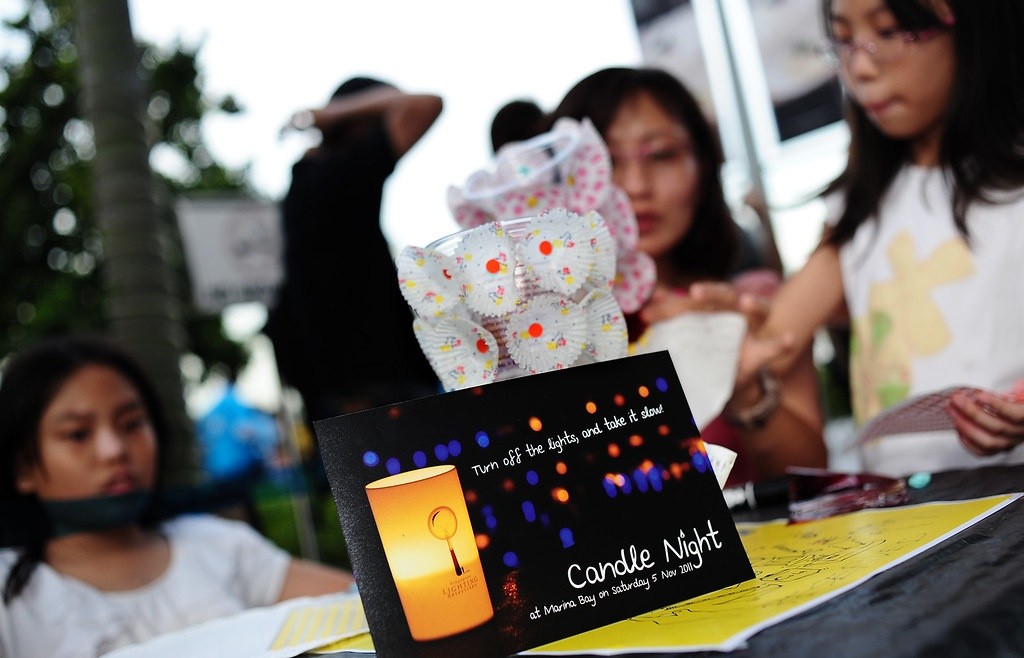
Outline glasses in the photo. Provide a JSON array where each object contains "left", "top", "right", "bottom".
[{"left": 813, "top": 19, "right": 953, "bottom": 68}]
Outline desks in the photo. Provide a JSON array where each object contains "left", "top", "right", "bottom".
[{"left": 102, "top": 460, "right": 1024, "bottom": 658}]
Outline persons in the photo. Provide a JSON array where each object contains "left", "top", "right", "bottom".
[
  {"left": 0, "top": 338, "right": 351, "bottom": 658},
  {"left": 187, "top": 364, "right": 278, "bottom": 538},
  {"left": 265, "top": 78, "right": 445, "bottom": 424},
  {"left": 489, "top": 0, "right": 1024, "bottom": 478}
]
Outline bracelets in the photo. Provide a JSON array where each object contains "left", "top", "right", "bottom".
[{"left": 727, "top": 372, "right": 781, "bottom": 427}]
